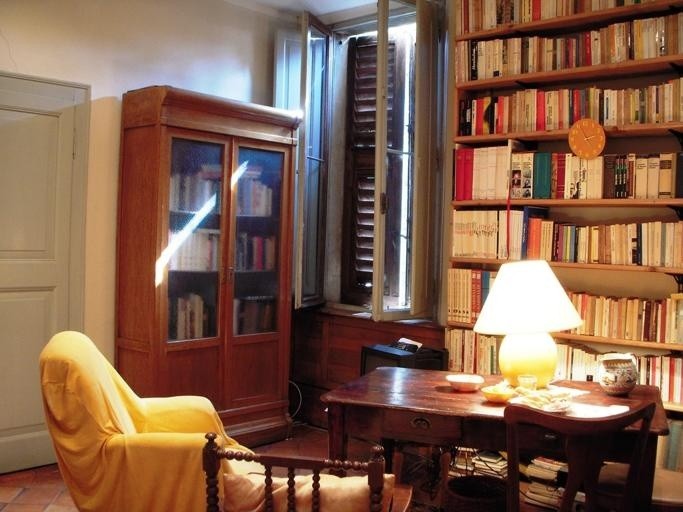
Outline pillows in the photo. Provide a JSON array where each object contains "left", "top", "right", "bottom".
[{"left": 223, "top": 470, "right": 396, "bottom": 512}]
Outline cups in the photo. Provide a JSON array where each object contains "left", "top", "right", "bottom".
[{"left": 518, "top": 375, "right": 538, "bottom": 391}]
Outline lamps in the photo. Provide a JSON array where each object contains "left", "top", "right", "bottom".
[{"left": 472, "top": 257, "right": 583, "bottom": 390}]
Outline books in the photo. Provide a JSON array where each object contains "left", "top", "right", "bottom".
[
  {"left": 446, "top": 208, "right": 683, "bottom": 344},
  {"left": 168, "top": 164, "right": 277, "bottom": 340},
  {"left": 455, "top": 0, "right": 683, "bottom": 86},
  {"left": 453, "top": 78, "right": 683, "bottom": 200},
  {"left": 446, "top": 328, "right": 683, "bottom": 405},
  {"left": 448, "top": 445, "right": 587, "bottom": 512}
]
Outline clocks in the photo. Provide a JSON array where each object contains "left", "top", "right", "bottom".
[{"left": 567, "top": 118, "right": 606, "bottom": 160}]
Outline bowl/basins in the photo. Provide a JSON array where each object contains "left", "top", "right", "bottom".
[
  {"left": 481, "top": 386, "right": 513, "bottom": 403},
  {"left": 446, "top": 374, "right": 484, "bottom": 391}
]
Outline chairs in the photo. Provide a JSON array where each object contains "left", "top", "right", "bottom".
[
  {"left": 201, "top": 431, "right": 414, "bottom": 512},
  {"left": 39, "top": 329, "right": 232, "bottom": 512},
  {"left": 504, "top": 399, "right": 657, "bottom": 512}
]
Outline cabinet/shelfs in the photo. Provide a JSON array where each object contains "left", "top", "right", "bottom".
[
  {"left": 446, "top": 0, "right": 683, "bottom": 511},
  {"left": 114, "top": 84, "right": 304, "bottom": 446}
]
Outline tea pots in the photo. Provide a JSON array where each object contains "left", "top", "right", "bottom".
[{"left": 596, "top": 353, "right": 639, "bottom": 396}]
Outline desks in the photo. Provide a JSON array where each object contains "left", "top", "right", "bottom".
[{"left": 319, "top": 365, "right": 669, "bottom": 512}]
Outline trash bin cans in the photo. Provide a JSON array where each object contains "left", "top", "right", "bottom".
[{"left": 444, "top": 475, "right": 507, "bottom": 512}]
